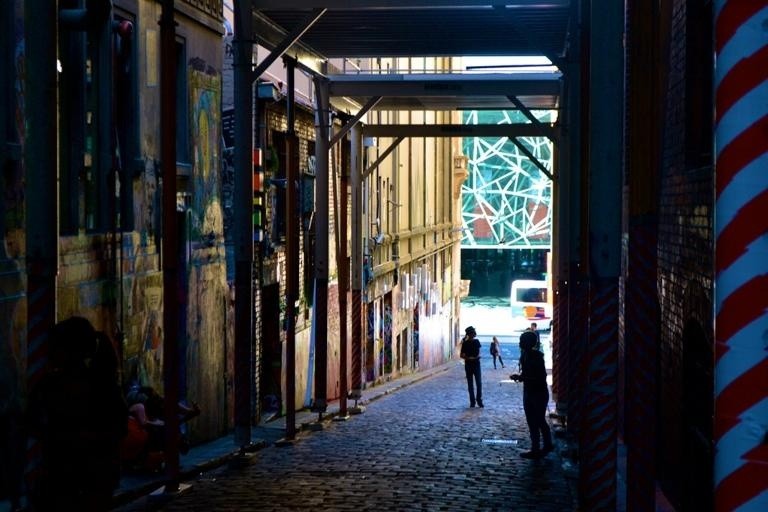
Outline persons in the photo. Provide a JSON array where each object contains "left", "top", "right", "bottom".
[
  {"left": 93, "top": 328, "right": 205, "bottom": 481},
  {"left": 460, "top": 325, "right": 485, "bottom": 408},
  {"left": 508, "top": 331, "right": 553, "bottom": 459},
  {"left": 530, "top": 322, "right": 540, "bottom": 352},
  {"left": 16, "top": 316, "right": 131, "bottom": 512},
  {"left": 489, "top": 336, "right": 507, "bottom": 371}
]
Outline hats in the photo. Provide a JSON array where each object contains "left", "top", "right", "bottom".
[{"left": 464, "top": 326, "right": 474, "bottom": 335}]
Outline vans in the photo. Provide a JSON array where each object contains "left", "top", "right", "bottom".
[{"left": 510, "top": 279, "right": 553, "bottom": 334}]
